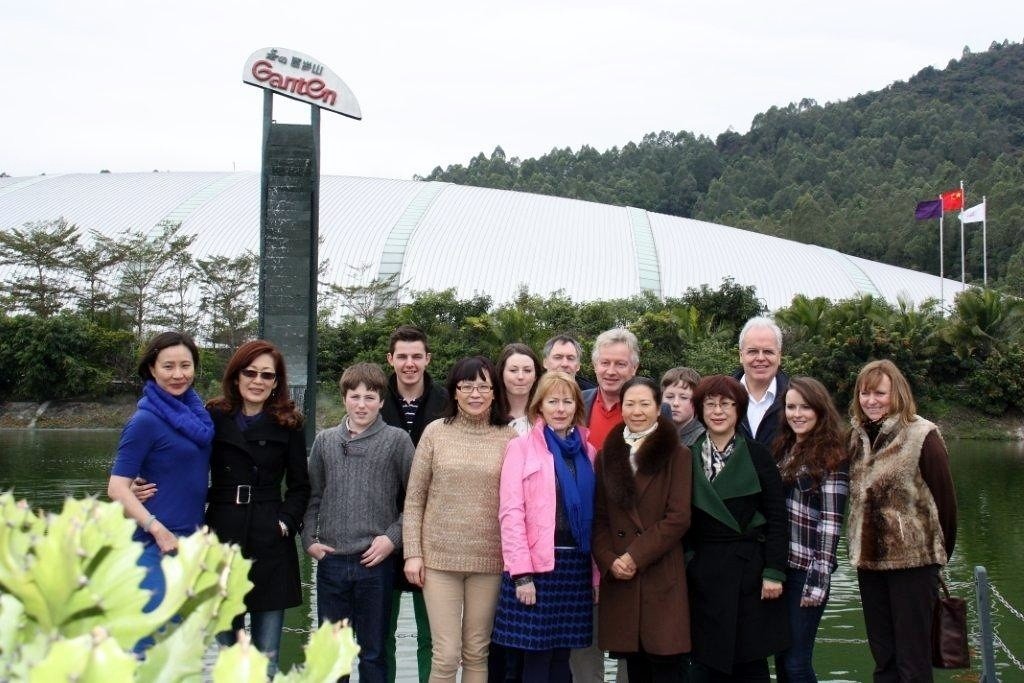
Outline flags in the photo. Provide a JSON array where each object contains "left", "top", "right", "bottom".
[
  {"left": 914, "top": 200, "right": 944, "bottom": 220},
  {"left": 958, "top": 202, "right": 986, "bottom": 223},
  {"left": 940, "top": 188, "right": 964, "bottom": 210}
]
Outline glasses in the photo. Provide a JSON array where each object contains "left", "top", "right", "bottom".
[
  {"left": 240, "top": 369, "right": 277, "bottom": 380},
  {"left": 456, "top": 383, "right": 495, "bottom": 393},
  {"left": 702, "top": 401, "right": 736, "bottom": 408}
]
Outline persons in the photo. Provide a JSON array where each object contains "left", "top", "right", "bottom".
[
  {"left": 299, "top": 361, "right": 416, "bottom": 683},
  {"left": 844, "top": 360, "right": 958, "bottom": 683},
  {"left": 108, "top": 332, "right": 215, "bottom": 661},
  {"left": 379, "top": 316, "right": 849, "bottom": 683},
  {"left": 130, "top": 340, "right": 310, "bottom": 683}
]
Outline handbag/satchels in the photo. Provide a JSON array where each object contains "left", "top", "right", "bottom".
[{"left": 932, "top": 574, "right": 970, "bottom": 669}]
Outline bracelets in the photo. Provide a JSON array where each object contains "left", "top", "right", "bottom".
[
  {"left": 278, "top": 519, "right": 289, "bottom": 536},
  {"left": 514, "top": 575, "right": 534, "bottom": 587},
  {"left": 144, "top": 514, "right": 156, "bottom": 532}
]
